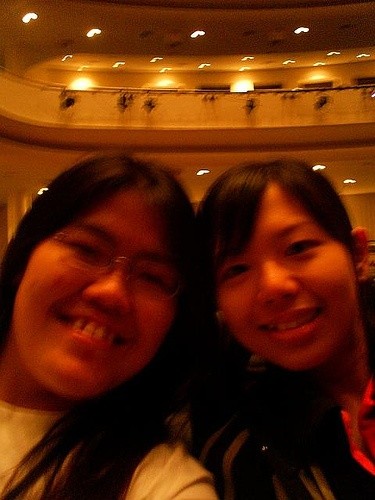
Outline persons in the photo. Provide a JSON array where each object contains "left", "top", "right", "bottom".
[
  {"left": 0, "top": 150, "right": 220, "bottom": 499},
  {"left": 195, "top": 157, "right": 375, "bottom": 500}
]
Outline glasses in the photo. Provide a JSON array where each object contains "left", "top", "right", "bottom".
[{"left": 49, "top": 229, "right": 181, "bottom": 300}]
